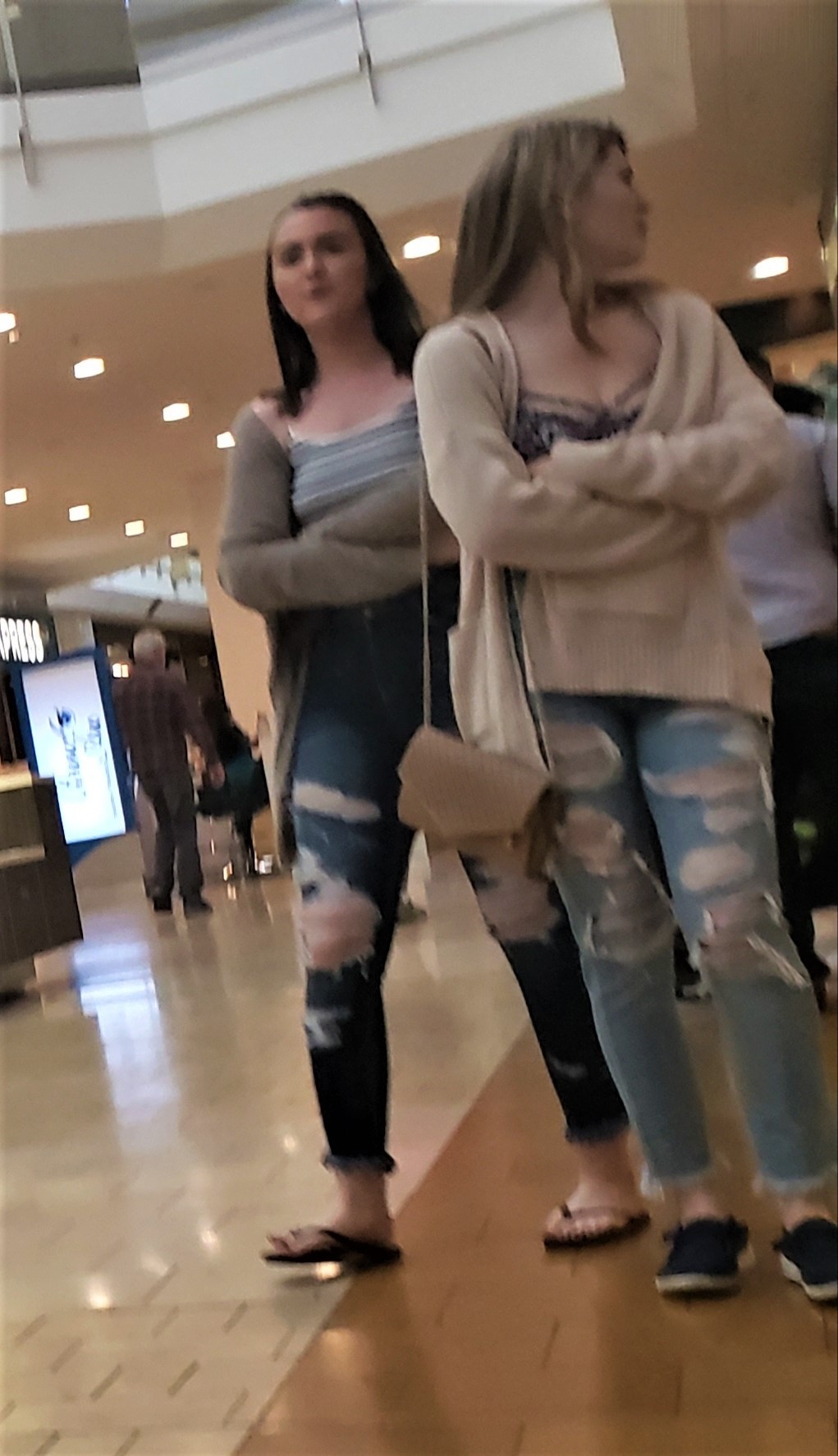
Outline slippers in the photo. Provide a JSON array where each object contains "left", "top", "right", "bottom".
[
  {"left": 263, "top": 1229, "right": 399, "bottom": 1264},
  {"left": 544, "top": 1202, "right": 651, "bottom": 1249}
]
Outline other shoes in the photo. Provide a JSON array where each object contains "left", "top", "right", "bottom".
[
  {"left": 183, "top": 900, "right": 210, "bottom": 915},
  {"left": 150, "top": 885, "right": 173, "bottom": 916}
]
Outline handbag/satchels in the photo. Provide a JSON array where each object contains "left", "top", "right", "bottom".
[{"left": 394, "top": 725, "right": 559, "bottom": 876}]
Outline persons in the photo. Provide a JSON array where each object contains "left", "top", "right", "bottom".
[
  {"left": 410, "top": 114, "right": 838, "bottom": 1298},
  {"left": 196, "top": 690, "right": 270, "bottom": 881},
  {"left": 109, "top": 627, "right": 224, "bottom": 916},
  {"left": 214, "top": 190, "right": 650, "bottom": 1263}
]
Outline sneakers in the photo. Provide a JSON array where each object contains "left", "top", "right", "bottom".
[
  {"left": 653, "top": 1213, "right": 753, "bottom": 1291},
  {"left": 772, "top": 1216, "right": 838, "bottom": 1300}
]
